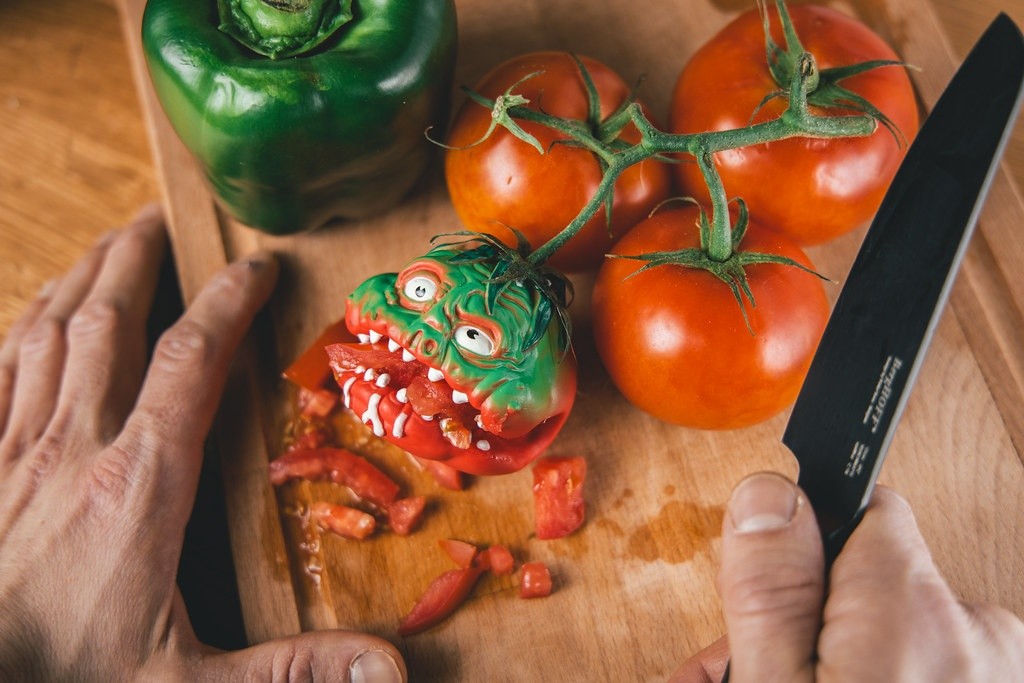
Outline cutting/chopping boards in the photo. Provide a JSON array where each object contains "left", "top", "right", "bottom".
[{"left": 118, "top": 0, "right": 1024, "bottom": 682}]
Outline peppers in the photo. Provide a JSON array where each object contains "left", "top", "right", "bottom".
[{"left": 141, "top": 0, "right": 460, "bottom": 234}]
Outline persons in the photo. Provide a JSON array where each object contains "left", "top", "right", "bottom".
[{"left": 0, "top": 205, "right": 1024, "bottom": 682}]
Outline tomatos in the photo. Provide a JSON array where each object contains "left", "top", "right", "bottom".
[
  {"left": 449, "top": 48, "right": 665, "bottom": 271},
  {"left": 672, "top": 3, "right": 918, "bottom": 247},
  {"left": 592, "top": 208, "right": 831, "bottom": 427}
]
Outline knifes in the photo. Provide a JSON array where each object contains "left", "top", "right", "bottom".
[{"left": 722, "top": 12, "right": 1024, "bottom": 682}]
